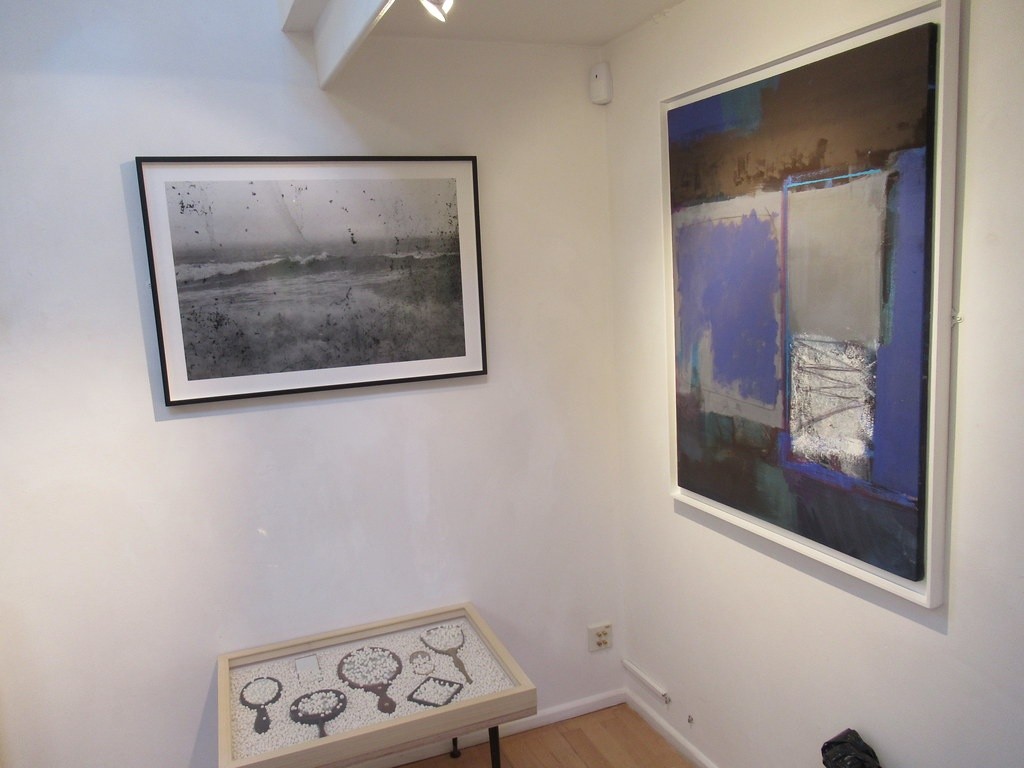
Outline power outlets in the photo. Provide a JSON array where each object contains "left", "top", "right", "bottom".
[{"left": 587, "top": 623, "right": 612, "bottom": 651}]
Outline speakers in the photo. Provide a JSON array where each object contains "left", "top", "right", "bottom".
[{"left": 590, "top": 62, "right": 613, "bottom": 104}]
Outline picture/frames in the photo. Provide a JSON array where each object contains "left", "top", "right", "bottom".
[
  {"left": 134, "top": 155, "right": 488, "bottom": 406},
  {"left": 660, "top": 0, "right": 962, "bottom": 610}
]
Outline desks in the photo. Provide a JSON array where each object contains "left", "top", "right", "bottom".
[{"left": 217, "top": 602, "right": 537, "bottom": 768}]
